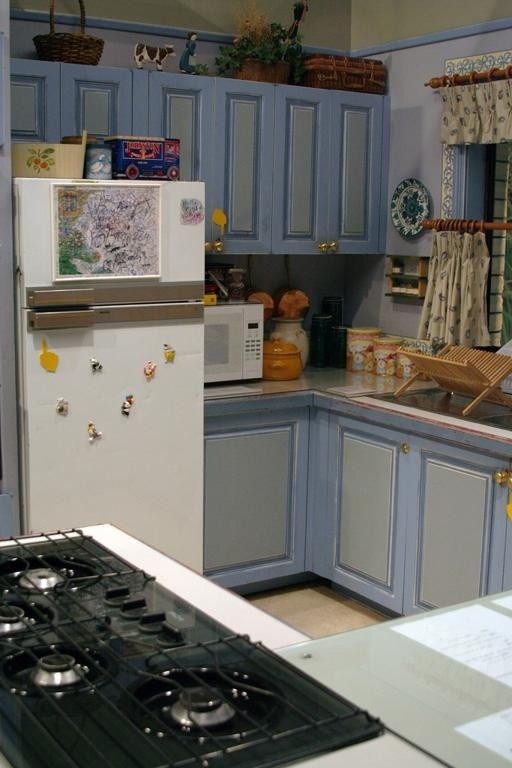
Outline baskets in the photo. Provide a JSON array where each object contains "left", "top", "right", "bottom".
[
  {"left": 33, "top": 0, "right": 104, "bottom": 66},
  {"left": 233, "top": 57, "right": 289, "bottom": 85}
]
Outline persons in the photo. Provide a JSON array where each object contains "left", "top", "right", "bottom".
[{"left": 179, "top": 31, "right": 200, "bottom": 75}]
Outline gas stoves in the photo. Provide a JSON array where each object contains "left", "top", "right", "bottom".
[{"left": 0, "top": 529, "right": 385, "bottom": 768}]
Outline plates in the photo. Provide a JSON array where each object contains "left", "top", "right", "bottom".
[{"left": 390, "top": 178, "right": 432, "bottom": 241}]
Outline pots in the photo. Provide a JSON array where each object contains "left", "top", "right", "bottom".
[{"left": 263, "top": 332, "right": 303, "bottom": 381}]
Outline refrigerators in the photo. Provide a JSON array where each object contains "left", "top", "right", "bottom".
[{"left": 8, "top": 178, "right": 205, "bottom": 582}]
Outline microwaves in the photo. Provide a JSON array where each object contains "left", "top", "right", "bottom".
[{"left": 205, "top": 303, "right": 264, "bottom": 386}]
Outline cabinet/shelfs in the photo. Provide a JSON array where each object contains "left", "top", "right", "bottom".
[
  {"left": 274, "top": 83, "right": 391, "bottom": 256},
  {"left": 148, "top": 70, "right": 277, "bottom": 254},
  {"left": 304, "top": 391, "right": 512, "bottom": 616},
  {"left": 8, "top": 58, "right": 147, "bottom": 138},
  {"left": 204, "top": 392, "right": 312, "bottom": 587}
]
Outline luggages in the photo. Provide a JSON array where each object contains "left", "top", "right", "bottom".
[{"left": 303, "top": 54, "right": 385, "bottom": 94}]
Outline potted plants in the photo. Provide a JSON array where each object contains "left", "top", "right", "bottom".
[{"left": 192, "top": 18, "right": 309, "bottom": 85}]
